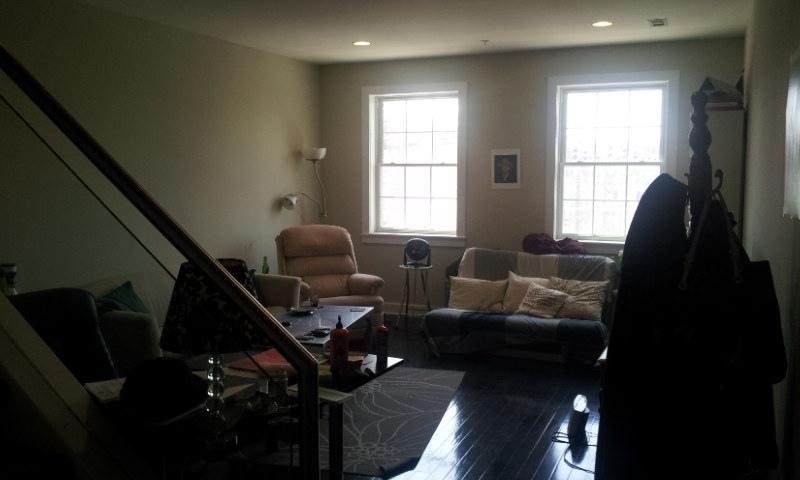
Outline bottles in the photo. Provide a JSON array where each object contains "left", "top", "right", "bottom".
[
  {"left": 329, "top": 315, "right": 350, "bottom": 368},
  {"left": 259, "top": 371, "right": 269, "bottom": 394},
  {"left": 375, "top": 321, "right": 389, "bottom": 362},
  {"left": 262, "top": 256, "right": 269, "bottom": 274}
]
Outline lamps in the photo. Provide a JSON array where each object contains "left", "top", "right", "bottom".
[{"left": 281, "top": 147, "right": 328, "bottom": 224}]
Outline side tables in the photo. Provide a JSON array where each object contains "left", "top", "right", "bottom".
[{"left": 396, "top": 262, "right": 432, "bottom": 336}]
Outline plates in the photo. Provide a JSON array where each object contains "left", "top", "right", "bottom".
[{"left": 290, "top": 307, "right": 317, "bottom": 314}]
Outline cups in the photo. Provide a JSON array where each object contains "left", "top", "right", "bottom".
[
  {"left": 574, "top": 394, "right": 587, "bottom": 411},
  {"left": 310, "top": 295, "right": 320, "bottom": 306},
  {"left": 0, "top": 262, "right": 18, "bottom": 295}
]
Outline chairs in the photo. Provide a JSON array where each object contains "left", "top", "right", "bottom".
[
  {"left": 7, "top": 288, "right": 119, "bottom": 382},
  {"left": 0, "top": 291, "right": 260, "bottom": 480},
  {"left": 275, "top": 224, "right": 384, "bottom": 341}
]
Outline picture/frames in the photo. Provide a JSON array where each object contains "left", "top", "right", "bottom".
[{"left": 491, "top": 148, "right": 521, "bottom": 189}]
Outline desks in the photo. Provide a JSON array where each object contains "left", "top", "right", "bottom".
[{"left": 186, "top": 352, "right": 404, "bottom": 480}]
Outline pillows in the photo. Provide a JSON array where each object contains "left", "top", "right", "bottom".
[
  {"left": 99, "top": 281, "right": 150, "bottom": 313},
  {"left": 448, "top": 270, "right": 610, "bottom": 320}
]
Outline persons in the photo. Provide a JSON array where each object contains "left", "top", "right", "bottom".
[{"left": 498, "top": 157, "right": 514, "bottom": 183}]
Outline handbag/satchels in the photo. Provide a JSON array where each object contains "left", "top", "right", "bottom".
[{"left": 614, "top": 262, "right": 786, "bottom": 385}]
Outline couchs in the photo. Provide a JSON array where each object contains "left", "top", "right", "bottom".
[
  {"left": 422, "top": 247, "right": 617, "bottom": 368},
  {"left": 86, "top": 268, "right": 301, "bottom": 370}
]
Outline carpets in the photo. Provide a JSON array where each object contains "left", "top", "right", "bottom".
[{"left": 231, "top": 351, "right": 474, "bottom": 480}]
